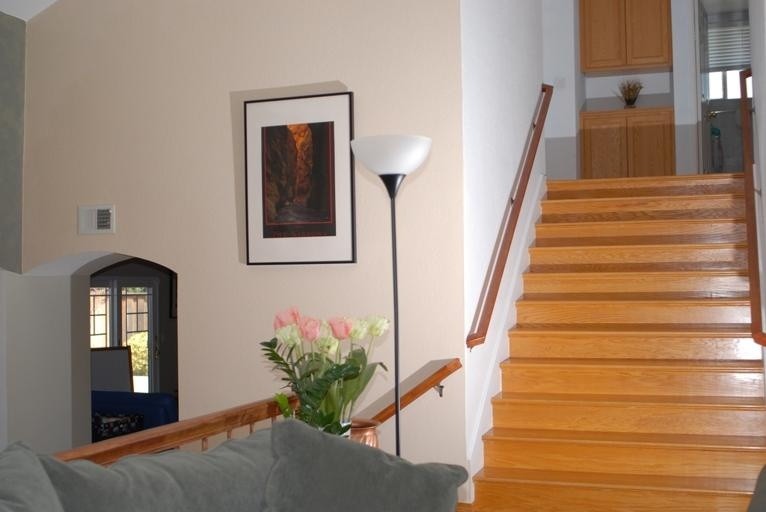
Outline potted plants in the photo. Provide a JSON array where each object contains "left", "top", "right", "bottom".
[{"left": 619, "top": 78, "right": 643, "bottom": 108}]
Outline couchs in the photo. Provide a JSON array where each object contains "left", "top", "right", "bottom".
[{"left": 0, "top": 418, "right": 470, "bottom": 512}]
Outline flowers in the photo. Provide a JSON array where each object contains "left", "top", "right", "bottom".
[{"left": 261, "top": 306, "right": 390, "bottom": 436}]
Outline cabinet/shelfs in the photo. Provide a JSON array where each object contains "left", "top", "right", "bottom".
[
  {"left": 579, "top": 0, "right": 672, "bottom": 77},
  {"left": 579, "top": 107, "right": 673, "bottom": 179}
]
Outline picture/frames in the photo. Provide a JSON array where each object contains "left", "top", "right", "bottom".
[{"left": 243, "top": 91, "right": 356, "bottom": 265}]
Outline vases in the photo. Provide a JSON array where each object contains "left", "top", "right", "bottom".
[
  {"left": 349, "top": 418, "right": 381, "bottom": 448},
  {"left": 318, "top": 422, "right": 351, "bottom": 439}
]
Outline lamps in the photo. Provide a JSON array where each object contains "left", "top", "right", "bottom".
[{"left": 351, "top": 134, "right": 432, "bottom": 458}]
